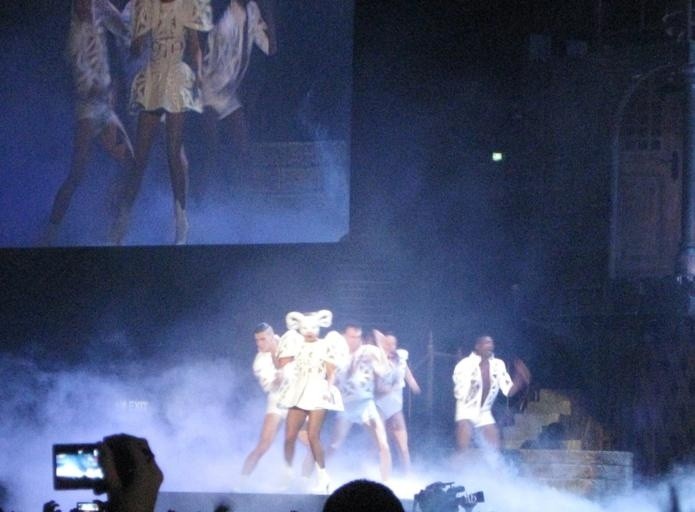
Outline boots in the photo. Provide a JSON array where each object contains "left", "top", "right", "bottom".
[
  {"left": 173, "top": 199, "right": 190, "bottom": 245},
  {"left": 314, "top": 460, "right": 329, "bottom": 496}
]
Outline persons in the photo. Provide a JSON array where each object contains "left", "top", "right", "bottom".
[
  {"left": 43, "top": 1, "right": 135, "bottom": 245},
  {"left": 105, "top": 1, "right": 214, "bottom": 246},
  {"left": 450, "top": 332, "right": 531, "bottom": 472},
  {"left": 193, "top": 0, "right": 278, "bottom": 212},
  {"left": 322, "top": 477, "right": 409, "bottom": 512},
  {"left": 240, "top": 308, "right": 421, "bottom": 482},
  {"left": 96, "top": 432, "right": 163, "bottom": 511}
]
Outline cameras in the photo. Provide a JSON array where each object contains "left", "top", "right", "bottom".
[
  {"left": 77, "top": 502, "right": 100, "bottom": 512},
  {"left": 53, "top": 444, "right": 105, "bottom": 490}
]
